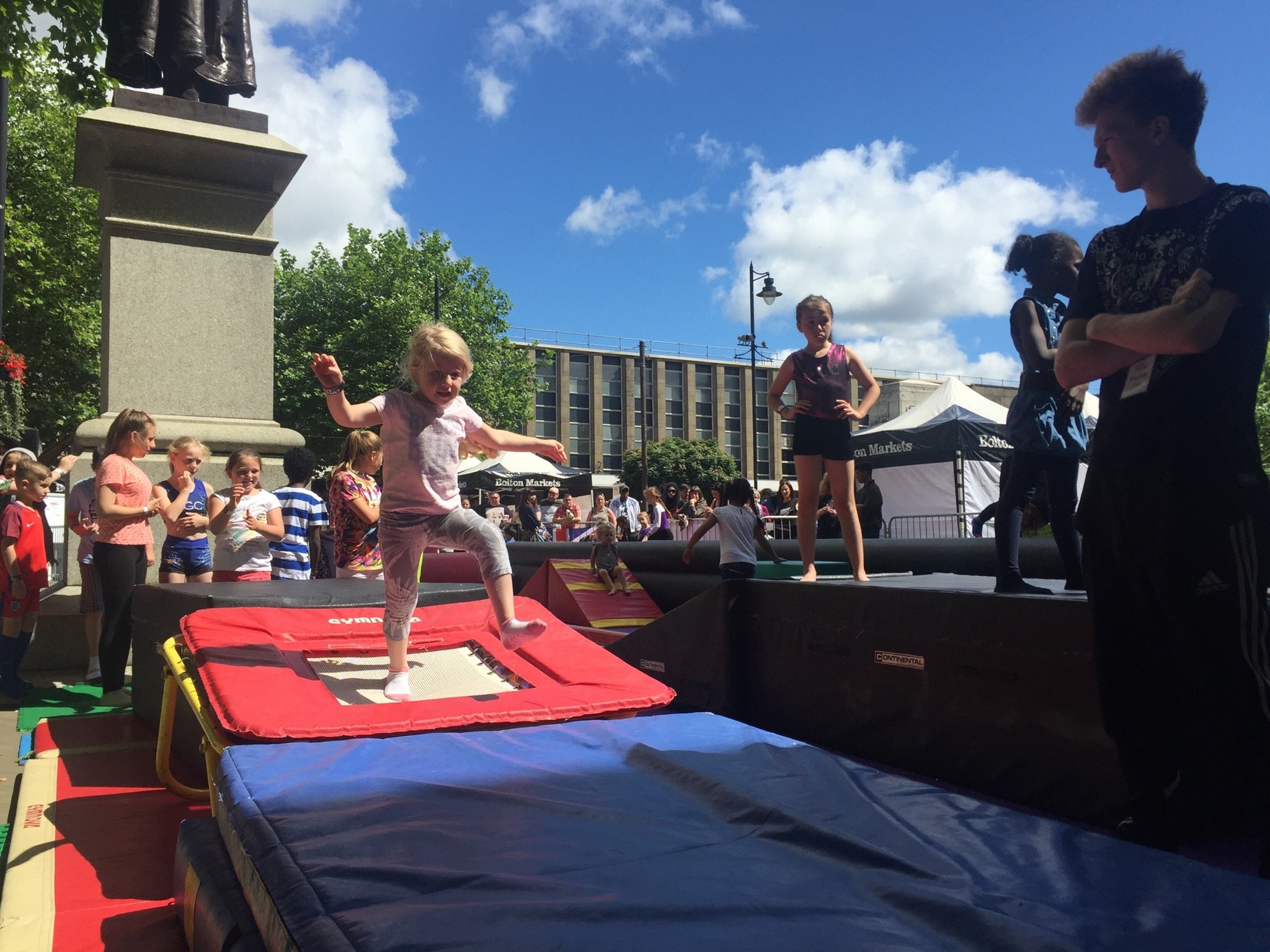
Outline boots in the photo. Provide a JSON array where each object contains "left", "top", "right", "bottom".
[{"left": 0, "top": 630, "right": 34, "bottom": 697}]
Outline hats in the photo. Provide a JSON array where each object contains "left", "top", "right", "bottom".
[
  {"left": 549, "top": 487, "right": 559, "bottom": 494},
  {"left": 0, "top": 447, "right": 37, "bottom": 471},
  {"left": 620, "top": 485, "right": 630, "bottom": 491}
]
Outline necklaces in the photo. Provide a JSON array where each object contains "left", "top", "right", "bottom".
[{"left": 607, "top": 553, "right": 610, "bottom": 556}]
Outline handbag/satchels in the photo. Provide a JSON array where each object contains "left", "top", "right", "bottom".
[{"left": 529, "top": 531, "right": 545, "bottom": 543}]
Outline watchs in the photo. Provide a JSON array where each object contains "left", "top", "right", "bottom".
[{"left": 776, "top": 405, "right": 786, "bottom": 415}]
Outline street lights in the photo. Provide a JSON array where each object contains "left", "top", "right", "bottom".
[{"left": 735, "top": 261, "right": 783, "bottom": 491}]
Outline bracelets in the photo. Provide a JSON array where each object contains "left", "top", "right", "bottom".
[
  {"left": 224, "top": 506, "right": 234, "bottom": 516},
  {"left": 143, "top": 506, "right": 148, "bottom": 516},
  {"left": 321, "top": 380, "right": 346, "bottom": 397},
  {"left": 55, "top": 466, "right": 68, "bottom": 477},
  {"left": 9, "top": 574, "right": 23, "bottom": 581}
]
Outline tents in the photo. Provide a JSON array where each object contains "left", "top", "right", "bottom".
[
  {"left": 850, "top": 373, "right": 1106, "bottom": 542},
  {"left": 457, "top": 451, "right": 621, "bottom": 517}
]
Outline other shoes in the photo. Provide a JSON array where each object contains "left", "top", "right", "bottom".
[
  {"left": 679, "top": 514, "right": 688, "bottom": 531},
  {"left": 100, "top": 687, "right": 133, "bottom": 706},
  {"left": 86, "top": 668, "right": 103, "bottom": 686}
]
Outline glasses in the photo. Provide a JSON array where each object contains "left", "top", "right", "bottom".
[
  {"left": 491, "top": 497, "right": 498, "bottom": 499},
  {"left": 667, "top": 489, "right": 676, "bottom": 494}
]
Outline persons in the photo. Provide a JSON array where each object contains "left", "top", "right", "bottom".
[
  {"left": 307, "top": 324, "right": 569, "bottom": 686},
  {"left": 589, "top": 521, "right": 632, "bottom": 596},
  {"left": 970, "top": 46, "right": 1270, "bottom": 874},
  {"left": 765, "top": 295, "right": 881, "bottom": 584},
  {"left": 0, "top": 409, "right": 889, "bottom": 707}
]
